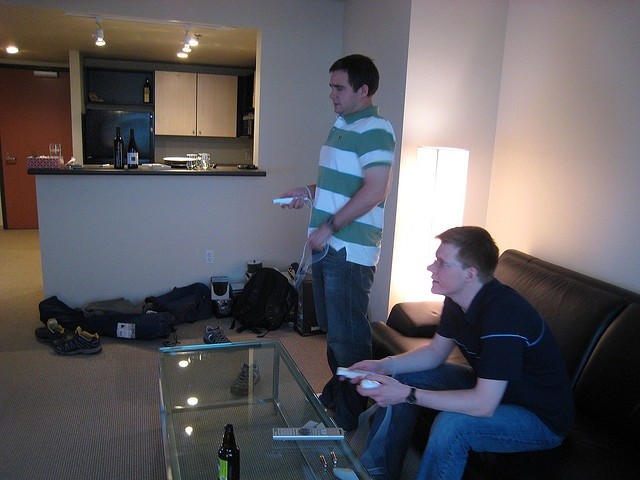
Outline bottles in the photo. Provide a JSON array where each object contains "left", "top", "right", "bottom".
[
  {"left": 127, "top": 128, "right": 138, "bottom": 168},
  {"left": 218, "top": 423, "right": 240, "bottom": 480},
  {"left": 114, "top": 127, "right": 125, "bottom": 169},
  {"left": 143, "top": 75, "right": 151, "bottom": 103}
]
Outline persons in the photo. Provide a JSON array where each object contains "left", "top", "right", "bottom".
[
  {"left": 279, "top": 54, "right": 396, "bottom": 431},
  {"left": 331, "top": 226, "right": 577, "bottom": 480}
]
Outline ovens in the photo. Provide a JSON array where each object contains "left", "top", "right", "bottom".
[{"left": 85, "top": 107, "right": 154, "bottom": 164}]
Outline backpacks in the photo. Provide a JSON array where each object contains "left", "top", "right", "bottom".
[{"left": 229, "top": 267, "right": 299, "bottom": 338}]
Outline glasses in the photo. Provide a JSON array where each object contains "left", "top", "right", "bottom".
[{"left": 436, "top": 260, "right": 464, "bottom": 270}]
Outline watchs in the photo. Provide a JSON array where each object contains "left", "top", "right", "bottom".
[
  {"left": 325, "top": 215, "right": 340, "bottom": 233},
  {"left": 407, "top": 388, "right": 417, "bottom": 405}
]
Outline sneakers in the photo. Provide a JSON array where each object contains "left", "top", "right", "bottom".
[
  {"left": 203, "top": 326, "right": 235, "bottom": 344},
  {"left": 333, "top": 466, "right": 359, "bottom": 480},
  {"left": 306, "top": 392, "right": 323, "bottom": 402},
  {"left": 34, "top": 318, "right": 67, "bottom": 343},
  {"left": 230, "top": 362, "right": 261, "bottom": 393},
  {"left": 53, "top": 327, "right": 102, "bottom": 356}
]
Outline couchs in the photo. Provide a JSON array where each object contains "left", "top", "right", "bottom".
[{"left": 370, "top": 248, "right": 640, "bottom": 480}]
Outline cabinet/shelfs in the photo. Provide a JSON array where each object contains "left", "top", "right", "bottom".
[{"left": 154, "top": 71, "right": 236, "bottom": 137}]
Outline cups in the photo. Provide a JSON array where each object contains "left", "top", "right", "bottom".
[
  {"left": 49, "top": 143, "right": 62, "bottom": 157},
  {"left": 186, "top": 154, "right": 199, "bottom": 170},
  {"left": 199, "top": 153, "right": 211, "bottom": 170}
]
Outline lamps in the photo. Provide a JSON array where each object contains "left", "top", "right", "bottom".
[
  {"left": 94, "top": 18, "right": 106, "bottom": 46},
  {"left": 410, "top": 148, "right": 470, "bottom": 304},
  {"left": 176, "top": 27, "right": 196, "bottom": 58}
]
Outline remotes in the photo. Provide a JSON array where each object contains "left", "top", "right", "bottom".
[{"left": 271, "top": 427, "right": 346, "bottom": 443}]
